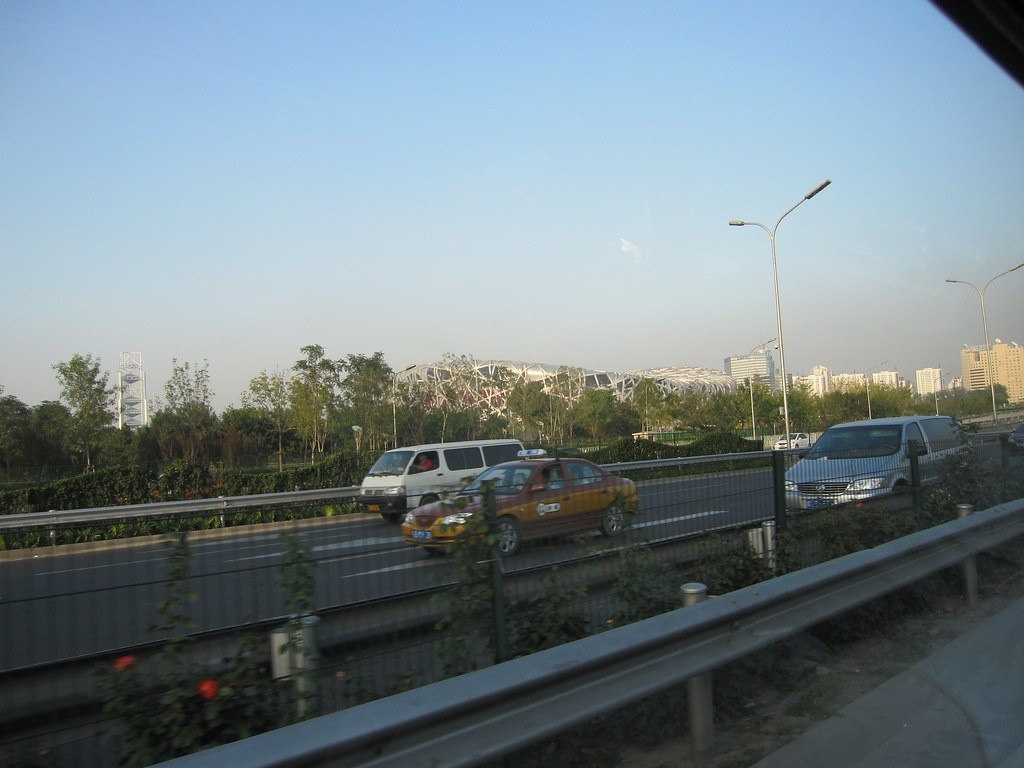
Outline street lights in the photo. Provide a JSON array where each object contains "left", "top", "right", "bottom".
[
  {"left": 865, "top": 360, "right": 888, "bottom": 420},
  {"left": 729, "top": 178, "right": 832, "bottom": 449},
  {"left": 393, "top": 364, "right": 416, "bottom": 449},
  {"left": 933, "top": 371, "right": 952, "bottom": 416},
  {"left": 748, "top": 337, "right": 776, "bottom": 441},
  {"left": 645, "top": 377, "right": 666, "bottom": 440},
  {"left": 945, "top": 263, "right": 1024, "bottom": 427}
]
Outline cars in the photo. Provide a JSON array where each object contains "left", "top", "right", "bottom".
[
  {"left": 402, "top": 449, "right": 639, "bottom": 558},
  {"left": 1007, "top": 422, "right": 1024, "bottom": 451},
  {"left": 775, "top": 433, "right": 812, "bottom": 450},
  {"left": 784, "top": 416, "right": 976, "bottom": 515}
]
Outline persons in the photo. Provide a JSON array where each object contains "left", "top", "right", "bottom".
[
  {"left": 531, "top": 466, "right": 590, "bottom": 489},
  {"left": 411, "top": 453, "right": 433, "bottom": 470}
]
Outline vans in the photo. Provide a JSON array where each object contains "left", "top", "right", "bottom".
[{"left": 358, "top": 439, "right": 529, "bottom": 522}]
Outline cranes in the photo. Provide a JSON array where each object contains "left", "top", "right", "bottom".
[{"left": 750, "top": 337, "right": 778, "bottom": 352}]
